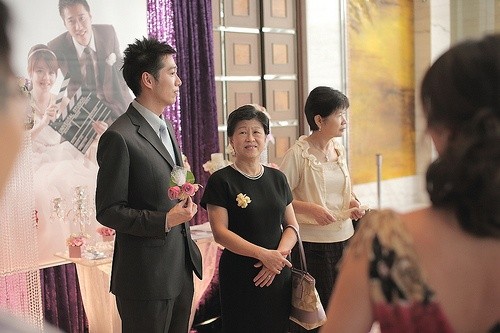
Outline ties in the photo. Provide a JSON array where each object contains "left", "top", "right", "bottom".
[
  {"left": 84, "top": 47, "right": 96, "bottom": 95},
  {"left": 160, "top": 119, "right": 176, "bottom": 165}
]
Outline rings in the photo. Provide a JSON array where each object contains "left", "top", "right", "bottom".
[
  {"left": 267, "top": 274, "right": 272, "bottom": 278},
  {"left": 355, "top": 218, "right": 358, "bottom": 221}
]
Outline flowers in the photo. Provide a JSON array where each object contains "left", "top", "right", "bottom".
[
  {"left": 105, "top": 52, "right": 116, "bottom": 66},
  {"left": 66, "top": 233, "right": 88, "bottom": 247},
  {"left": 96, "top": 227, "right": 115, "bottom": 236},
  {"left": 168, "top": 166, "right": 203, "bottom": 200},
  {"left": 236, "top": 193, "right": 252, "bottom": 208}
]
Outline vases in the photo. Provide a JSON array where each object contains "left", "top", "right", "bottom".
[
  {"left": 69, "top": 246, "right": 84, "bottom": 258},
  {"left": 103, "top": 235, "right": 115, "bottom": 242}
]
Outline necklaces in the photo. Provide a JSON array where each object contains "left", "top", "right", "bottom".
[
  {"left": 310, "top": 133, "right": 332, "bottom": 162},
  {"left": 233, "top": 161, "right": 265, "bottom": 181},
  {"left": 31, "top": 94, "right": 52, "bottom": 116}
]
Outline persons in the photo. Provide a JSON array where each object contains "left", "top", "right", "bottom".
[
  {"left": 0, "top": 0, "right": 134, "bottom": 278},
  {"left": 318, "top": 31, "right": 500, "bottom": 333},
  {"left": 95, "top": 36, "right": 203, "bottom": 333},
  {"left": 199, "top": 103, "right": 301, "bottom": 333},
  {"left": 275, "top": 86, "right": 366, "bottom": 332}
]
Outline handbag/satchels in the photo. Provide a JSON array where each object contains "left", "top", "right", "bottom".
[{"left": 283, "top": 225, "right": 327, "bottom": 330}]
